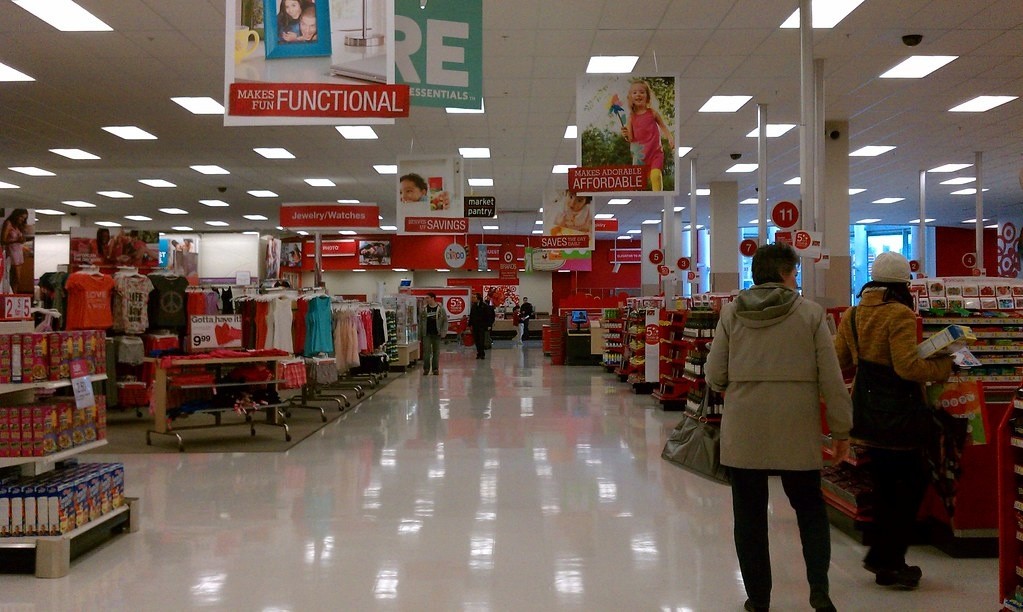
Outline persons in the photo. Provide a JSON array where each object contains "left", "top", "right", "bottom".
[
  {"left": 579, "top": 312, "right": 585, "bottom": 319},
  {"left": 512, "top": 305, "right": 525, "bottom": 345},
  {"left": 468, "top": 292, "right": 495, "bottom": 359},
  {"left": 520, "top": 297, "right": 536, "bottom": 341},
  {"left": 360, "top": 242, "right": 390, "bottom": 262},
  {"left": 1, "top": 209, "right": 30, "bottom": 288},
  {"left": 703, "top": 241, "right": 854, "bottom": 612},
  {"left": 554, "top": 189, "right": 592, "bottom": 232},
  {"left": 834, "top": 251, "right": 957, "bottom": 586},
  {"left": 623, "top": 87, "right": 675, "bottom": 192},
  {"left": 172, "top": 239, "right": 195, "bottom": 253},
  {"left": 418, "top": 292, "right": 448, "bottom": 376},
  {"left": 277, "top": 0, "right": 317, "bottom": 43},
  {"left": 400, "top": 174, "right": 428, "bottom": 203},
  {"left": 90, "top": 229, "right": 159, "bottom": 263}
]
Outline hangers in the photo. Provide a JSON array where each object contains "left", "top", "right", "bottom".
[
  {"left": 77, "top": 263, "right": 105, "bottom": 277},
  {"left": 148, "top": 266, "right": 181, "bottom": 279},
  {"left": 186, "top": 286, "right": 396, "bottom": 315},
  {"left": 116, "top": 265, "right": 146, "bottom": 278}
]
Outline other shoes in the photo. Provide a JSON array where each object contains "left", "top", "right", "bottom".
[
  {"left": 433, "top": 369, "right": 439, "bottom": 375},
  {"left": 744, "top": 598, "right": 769, "bottom": 612},
  {"left": 518, "top": 341, "right": 523, "bottom": 346},
  {"left": 423, "top": 369, "right": 429, "bottom": 375},
  {"left": 810, "top": 585, "right": 837, "bottom": 612},
  {"left": 512, "top": 338, "right": 516, "bottom": 342},
  {"left": 862, "top": 550, "right": 919, "bottom": 586},
  {"left": 476, "top": 351, "right": 485, "bottom": 360},
  {"left": 875, "top": 563, "right": 922, "bottom": 586}
]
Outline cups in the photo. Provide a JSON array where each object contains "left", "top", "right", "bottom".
[{"left": 234, "top": 26, "right": 260, "bottom": 65}]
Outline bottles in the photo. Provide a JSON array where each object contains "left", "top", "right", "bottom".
[
  {"left": 601, "top": 308, "right": 622, "bottom": 365},
  {"left": 683, "top": 317, "right": 724, "bottom": 418}
]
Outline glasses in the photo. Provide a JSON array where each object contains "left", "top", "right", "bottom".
[{"left": 906, "top": 281, "right": 910, "bottom": 286}]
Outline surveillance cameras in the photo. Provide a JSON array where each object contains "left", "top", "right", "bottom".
[
  {"left": 755, "top": 188, "right": 758, "bottom": 191},
  {"left": 827, "top": 129, "right": 840, "bottom": 139},
  {"left": 217, "top": 187, "right": 226, "bottom": 192},
  {"left": 902, "top": 35, "right": 922, "bottom": 46},
  {"left": 730, "top": 154, "right": 741, "bottom": 159},
  {"left": 71, "top": 212, "right": 77, "bottom": 215}
]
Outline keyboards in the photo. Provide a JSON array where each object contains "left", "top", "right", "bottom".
[{"left": 569, "top": 330, "right": 590, "bottom": 334}]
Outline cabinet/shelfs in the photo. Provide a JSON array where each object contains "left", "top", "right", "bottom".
[
  {"left": 0, "top": 323, "right": 142, "bottom": 581},
  {"left": 140, "top": 355, "right": 294, "bottom": 450},
  {"left": 602, "top": 297, "right": 1023, "bottom": 611}
]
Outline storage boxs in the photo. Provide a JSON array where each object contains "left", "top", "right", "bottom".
[{"left": 1, "top": 331, "right": 124, "bottom": 535}]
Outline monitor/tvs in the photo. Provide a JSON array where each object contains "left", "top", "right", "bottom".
[{"left": 571, "top": 310, "right": 587, "bottom": 330}]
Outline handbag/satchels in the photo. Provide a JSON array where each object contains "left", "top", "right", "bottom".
[
  {"left": 659, "top": 387, "right": 732, "bottom": 486},
  {"left": 850, "top": 360, "right": 917, "bottom": 443}
]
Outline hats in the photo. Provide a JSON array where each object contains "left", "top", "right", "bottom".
[{"left": 871, "top": 252, "right": 910, "bottom": 283}]
